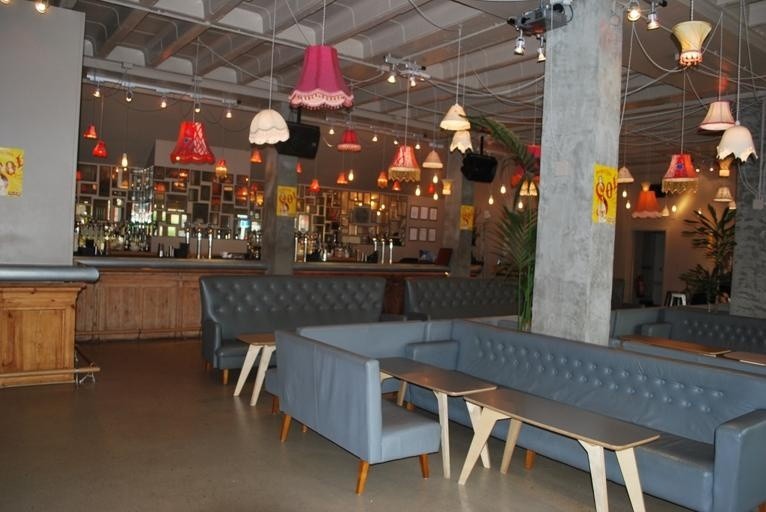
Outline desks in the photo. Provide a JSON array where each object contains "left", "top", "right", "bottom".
[
  {"left": 233, "top": 331, "right": 297, "bottom": 406},
  {"left": 375, "top": 358, "right": 497, "bottom": 479}
]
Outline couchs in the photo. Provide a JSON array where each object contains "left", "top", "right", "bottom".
[
  {"left": 200, "top": 274, "right": 407, "bottom": 385},
  {"left": 404, "top": 278, "right": 532, "bottom": 321}
]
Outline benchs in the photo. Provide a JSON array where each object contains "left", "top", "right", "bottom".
[
  {"left": 264, "top": 320, "right": 426, "bottom": 415},
  {"left": 274, "top": 328, "right": 442, "bottom": 495}
]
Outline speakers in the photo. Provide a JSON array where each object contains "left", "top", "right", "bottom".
[
  {"left": 276, "top": 120, "right": 320, "bottom": 160},
  {"left": 463, "top": 152, "right": 498, "bottom": 183}
]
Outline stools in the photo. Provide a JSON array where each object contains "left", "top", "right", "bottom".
[{"left": 664, "top": 291, "right": 687, "bottom": 308}]
